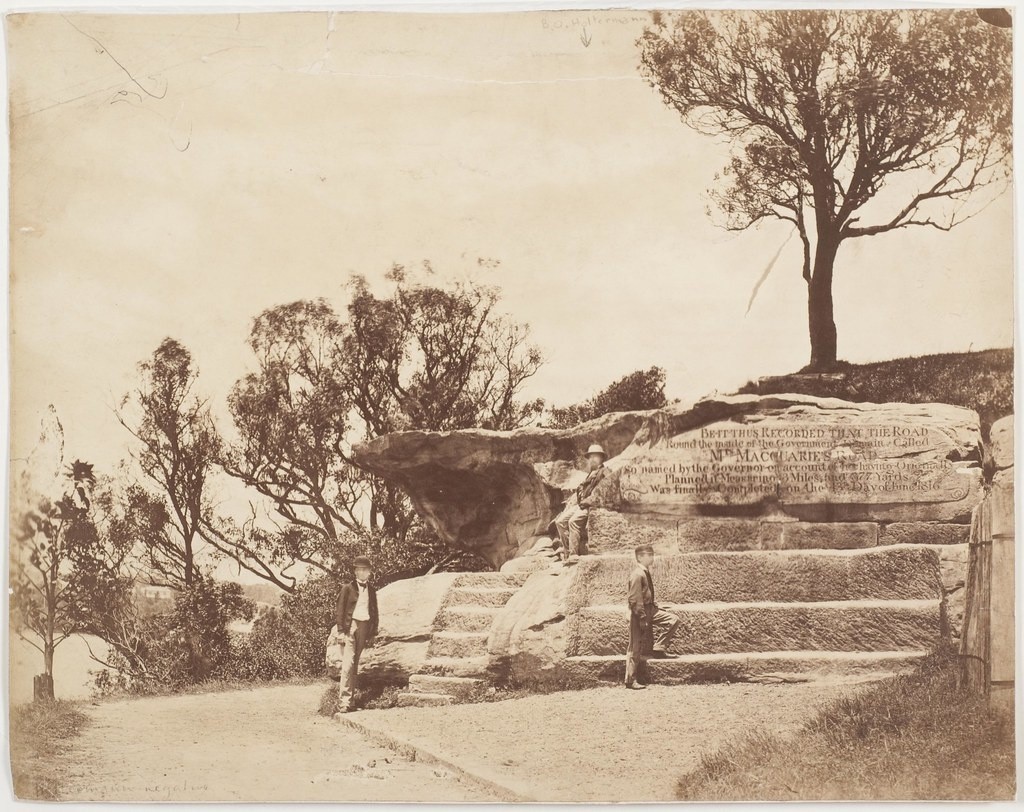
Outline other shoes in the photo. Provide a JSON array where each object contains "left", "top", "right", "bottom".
[
  {"left": 349, "top": 706, "right": 357, "bottom": 711},
  {"left": 654, "top": 650, "right": 677, "bottom": 659},
  {"left": 626, "top": 681, "right": 645, "bottom": 689},
  {"left": 340, "top": 706, "right": 348, "bottom": 713},
  {"left": 564, "top": 554, "right": 580, "bottom": 564}
]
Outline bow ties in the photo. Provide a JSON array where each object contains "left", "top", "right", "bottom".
[{"left": 356, "top": 580, "right": 368, "bottom": 589}]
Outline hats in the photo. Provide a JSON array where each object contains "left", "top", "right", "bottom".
[
  {"left": 352, "top": 556, "right": 372, "bottom": 568},
  {"left": 635, "top": 546, "right": 656, "bottom": 555},
  {"left": 584, "top": 445, "right": 608, "bottom": 460}
]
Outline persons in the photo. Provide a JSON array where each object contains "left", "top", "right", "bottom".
[
  {"left": 625, "top": 546, "right": 680, "bottom": 689},
  {"left": 555, "top": 444, "right": 607, "bottom": 565},
  {"left": 337, "top": 555, "right": 379, "bottom": 714}
]
